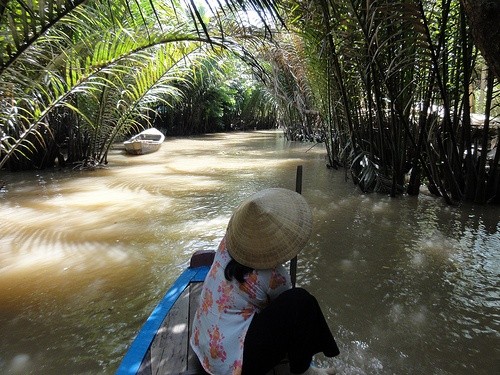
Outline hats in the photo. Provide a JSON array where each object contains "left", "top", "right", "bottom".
[{"left": 225, "top": 187, "right": 313, "bottom": 271}]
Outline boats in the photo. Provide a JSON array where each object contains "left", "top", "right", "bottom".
[{"left": 123, "top": 127, "right": 165, "bottom": 156}]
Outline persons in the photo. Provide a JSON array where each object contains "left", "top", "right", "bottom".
[{"left": 192, "top": 186, "right": 340, "bottom": 375}]
